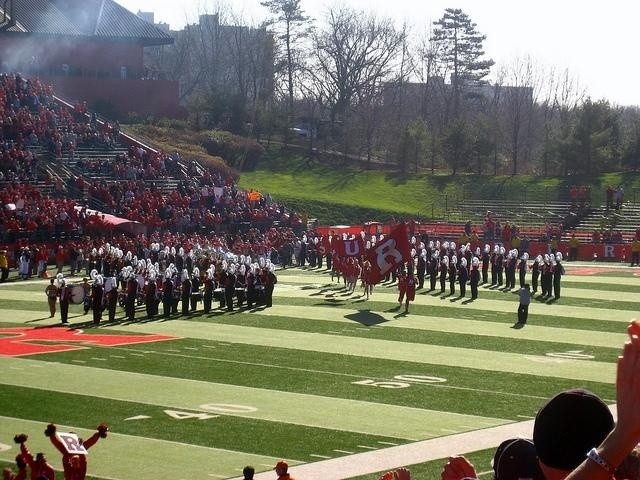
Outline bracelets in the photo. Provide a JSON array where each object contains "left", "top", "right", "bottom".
[{"left": 585, "top": 447, "right": 617, "bottom": 476}]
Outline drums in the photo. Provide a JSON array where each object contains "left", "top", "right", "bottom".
[
  {"left": 67, "top": 284, "right": 85, "bottom": 304},
  {"left": 192, "top": 291, "right": 202, "bottom": 302},
  {"left": 235, "top": 287, "right": 247, "bottom": 298},
  {"left": 213, "top": 288, "right": 224, "bottom": 300},
  {"left": 256, "top": 285, "right": 265, "bottom": 297}
]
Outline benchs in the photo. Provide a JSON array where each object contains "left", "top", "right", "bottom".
[
  {"left": 408, "top": 197, "right": 640, "bottom": 250},
  {"left": 58, "top": 148, "right": 185, "bottom": 188},
  {"left": 25, "top": 144, "right": 56, "bottom": 196}
]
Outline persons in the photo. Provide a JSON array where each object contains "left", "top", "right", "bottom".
[
  {"left": 13, "top": 452, "right": 29, "bottom": 480},
  {"left": 1, "top": 468, "right": 17, "bottom": 480},
  {"left": 560, "top": 318, "right": 640, "bottom": 480},
  {"left": 241, "top": 465, "right": 256, "bottom": 480},
  {"left": 272, "top": 461, "right": 296, "bottom": 480},
  {"left": 438, "top": 436, "right": 543, "bottom": 479},
  {"left": 531, "top": 386, "right": 613, "bottom": 480},
  {"left": 44, "top": 422, "right": 109, "bottom": 480},
  {"left": 613, "top": 452, "right": 640, "bottom": 480},
  {"left": 378, "top": 467, "right": 412, "bottom": 480},
  {"left": 14, "top": 432, "right": 56, "bottom": 480}
]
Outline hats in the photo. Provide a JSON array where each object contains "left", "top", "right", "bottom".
[
  {"left": 272, "top": 461, "right": 288, "bottom": 471},
  {"left": 410, "top": 235, "right": 564, "bottom": 268},
  {"left": 533, "top": 390, "right": 616, "bottom": 470},
  {"left": 303, "top": 231, "right": 384, "bottom": 248},
  {"left": 495, "top": 438, "right": 539, "bottom": 479},
  {"left": 56, "top": 242, "right": 273, "bottom": 285}
]
